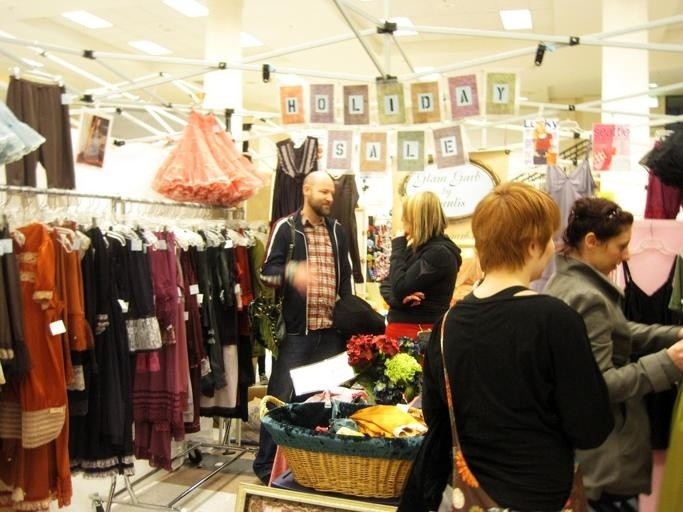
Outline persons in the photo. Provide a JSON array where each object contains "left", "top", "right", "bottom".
[
  {"left": 421, "top": 179, "right": 614, "bottom": 511},
  {"left": 379, "top": 191, "right": 462, "bottom": 342},
  {"left": 250, "top": 171, "right": 352, "bottom": 485},
  {"left": 541, "top": 195, "right": 683, "bottom": 511}
]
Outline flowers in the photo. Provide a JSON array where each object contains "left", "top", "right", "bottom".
[{"left": 346, "top": 328, "right": 423, "bottom": 406}]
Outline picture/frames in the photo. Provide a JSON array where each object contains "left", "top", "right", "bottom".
[{"left": 234, "top": 479, "right": 402, "bottom": 512}]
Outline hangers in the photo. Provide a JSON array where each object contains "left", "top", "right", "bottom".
[
  {"left": 1, "top": 190, "right": 271, "bottom": 252},
  {"left": 7, "top": 55, "right": 67, "bottom": 87}
]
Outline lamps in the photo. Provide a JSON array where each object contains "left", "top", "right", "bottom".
[
  {"left": 262, "top": 63, "right": 271, "bottom": 84},
  {"left": 533, "top": 39, "right": 547, "bottom": 66}
]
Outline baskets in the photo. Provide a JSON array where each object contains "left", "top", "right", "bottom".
[{"left": 259, "top": 395, "right": 425, "bottom": 500}]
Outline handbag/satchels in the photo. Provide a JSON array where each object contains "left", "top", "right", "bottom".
[{"left": 247, "top": 298, "right": 287, "bottom": 362}]
